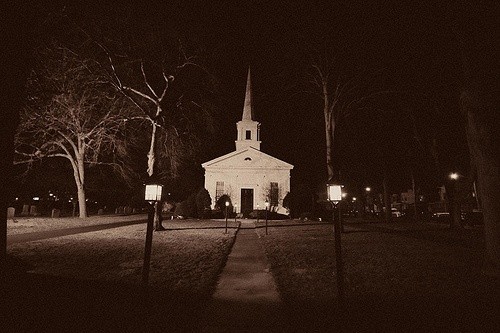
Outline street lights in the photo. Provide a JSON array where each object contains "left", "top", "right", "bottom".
[
  {"left": 325, "top": 172, "right": 344, "bottom": 306},
  {"left": 225, "top": 202, "right": 230, "bottom": 234},
  {"left": 134, "top": 169, "right": 165, "bottom": 293},
  {"left": 264, "top": 197, "right": 269, "bottom": 234}
]
